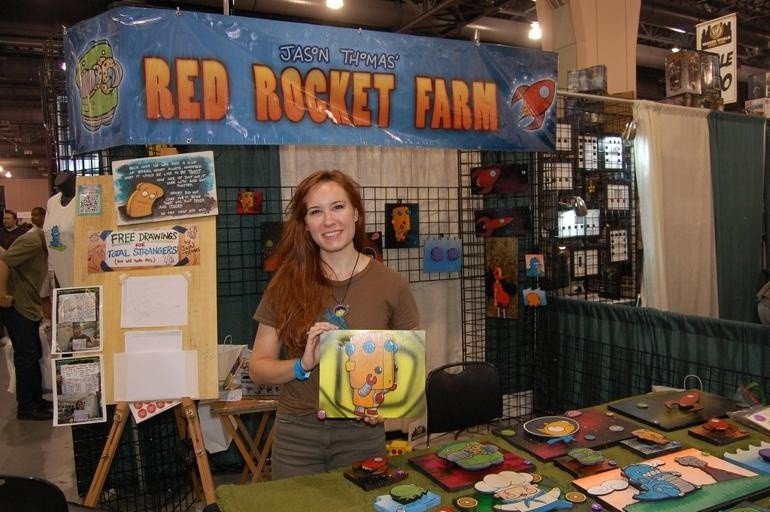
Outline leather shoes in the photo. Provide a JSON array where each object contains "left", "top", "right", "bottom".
[{"left": 16, "top": 398, "right": 53, "bottom": 421}]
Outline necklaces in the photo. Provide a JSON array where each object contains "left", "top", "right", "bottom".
[{"left": 318, "top": 251, "right": 360, "bottom": 320}]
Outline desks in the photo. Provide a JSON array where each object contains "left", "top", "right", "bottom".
[{"left": 212, "top": 383, "right": 770, "bottom": 510}]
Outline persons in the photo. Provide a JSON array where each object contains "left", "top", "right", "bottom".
[
  {"left": 64, "top": 401, "right": 91, "bottom": 422},
  {"left": 68, "top": 322, "right": 92, "bottom": 351},
  {"left": 42, "top": 170, "right": 76, "bottom": 288},
  {"left": 248, "top": 170, "right": 421, "bottom": 481},
  {"left": 0, "top": 207, "right": 53, "bottom": 420}
]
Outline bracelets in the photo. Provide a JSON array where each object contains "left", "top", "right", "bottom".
[{"left": 294, "top": 359, "right": 313, "bottom": 381}]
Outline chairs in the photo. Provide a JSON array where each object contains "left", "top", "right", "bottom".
[{"left": 423, "top": 356, "right": 505, "bottom": 451}]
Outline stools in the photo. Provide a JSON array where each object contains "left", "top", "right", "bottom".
[{"left": 204, "top": 394, "right": 280, "bottom": 484}]
